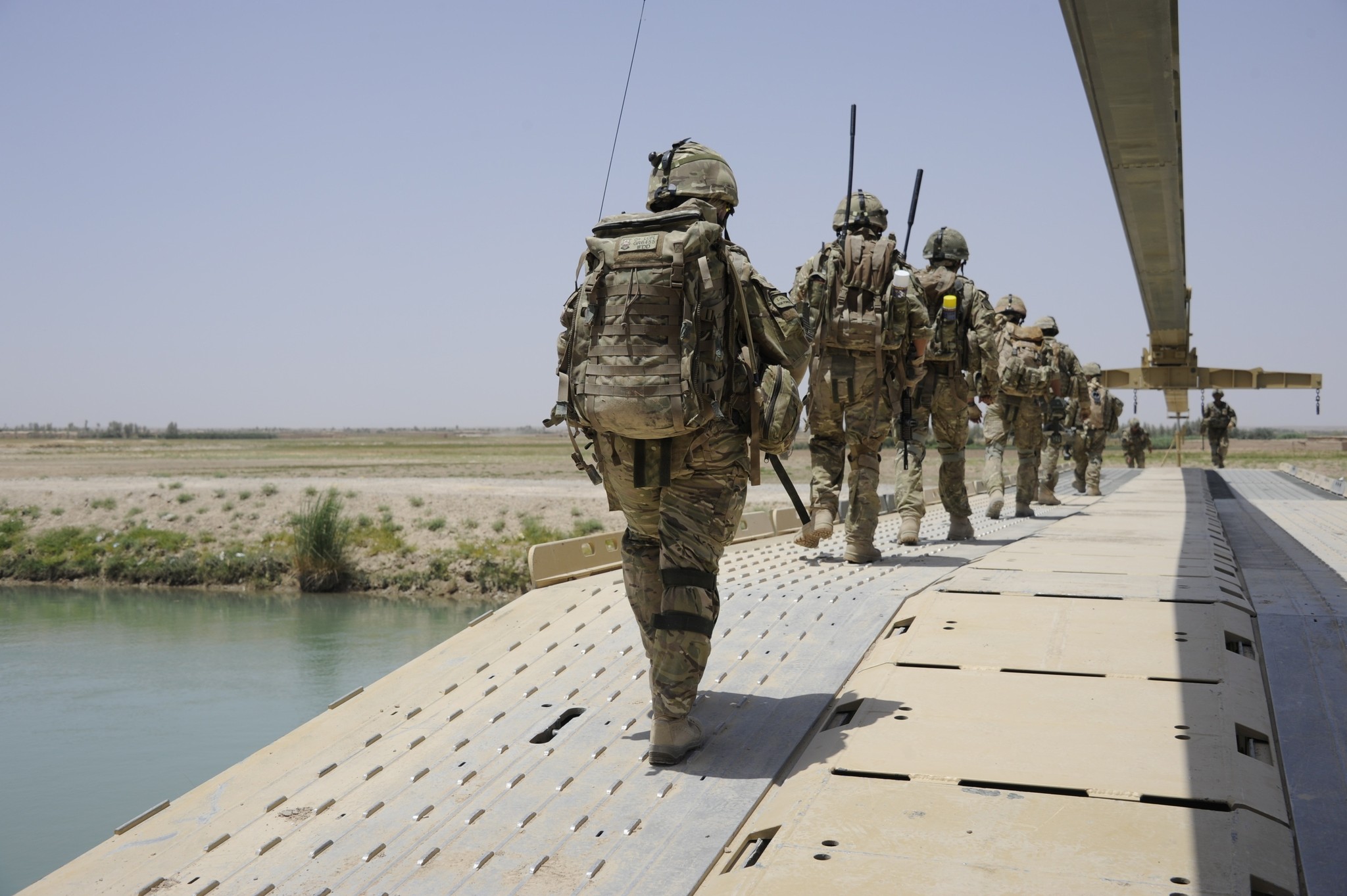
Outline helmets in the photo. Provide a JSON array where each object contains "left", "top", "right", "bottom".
[
  {"left": 923, "top": 227, "right": 969, "bottom": 260},
  {"left": 1083, "top": 363, "right": 1102, "bottom": 376},
  {"left": 1213, "top": 388, "right": 1224, "bottom": 398},
  {"left": 646, "top": 138, "right": 738, "bottom": 210},
  {"left": 1036, "top": 316, "right": 1059, "bottom": 336},
  {"left": 832, "top": 189, "right": 888, "bottom": 232},
  {"left": 1129, "top": 418, "right": 1139, "bottom": 428},
  {"left": 995, "top": 294, "right": 1026, "bottom": 318}
]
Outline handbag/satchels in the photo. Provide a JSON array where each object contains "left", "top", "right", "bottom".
[
  {"left": 745, "top": 363, "right": 803, "bottom": 454},
  {"left": 1109, "top": 397, "right": 1124, "bottom": 432}
]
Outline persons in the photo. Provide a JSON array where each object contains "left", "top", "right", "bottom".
[
  {"left": 1200, "top": 391, "right": 1237, "bottom": 469},
  {"left": 556, "top": 141, "right": 806, "bottom": 767},
  {"left": 1121, "top": 418, "right": 1152, "bottom": 468},
  {"left": 1063, "top": 362, "right": 1119, "bottom": 501},
  {"left": 790, "top": 191, "right": 935, "bottom": 562},
  {"left": 894, "top": 227, "right": 1004, "bottom": 543},
  {"left": 964, "top": 295, "right": 1092, "bottom": 519}
]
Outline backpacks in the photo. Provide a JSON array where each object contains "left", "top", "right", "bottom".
[
  {"left": 1075, "top": 383, "right": 1107, "bottom": 430},
  {"left": 803, "top": 232, "right": 910, "bottom": 351},
  {"left": 993, "top": 321, "right": 1053, "bottom": 398},
  {"left": 917, "top": 267, "right": 964, "bottom": 362},
  {"left": 562, "top": 199, "right": 731, "bottom": 440},
  {"left": 1209, "top": 403, "right": 1229, "bottom": 428}
]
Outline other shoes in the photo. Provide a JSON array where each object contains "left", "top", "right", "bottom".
[
  {"left": 1219, "top": 456, "right": 1224, "bottom": 468},
  {"left": 1213, "top": 459, "right": 1218, "bottom": 466}
]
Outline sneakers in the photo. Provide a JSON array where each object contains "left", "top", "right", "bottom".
[
  {"left": 986, "top": 490, "right": 1004, "bottom": 519},
  {"left": 897, "top": 517, "right": 921, "bottom": 543},
  {"left": 648, "top": 716, "right": 704, "bottom": 765},
  {"left": 794, "top": 509, "right": 834, "bottom": 548},
  {"left": 1072, "top": 480, "right": 1086, "bottom": 493},
  {"left": 844, "top": 541, "right": 881, "bottom": 562},
  {"left": 1037, "top": 483, "right": 1060, "bottom": 505},
  {"left": 948, "top": 517, "right": 974, "bottom": 539},
  {"left": 1085, "top": 484, "right": 1101, "bottom": 496},
  {"left": 1015, "top": 502, "right": 1034, "bottom": 517}
]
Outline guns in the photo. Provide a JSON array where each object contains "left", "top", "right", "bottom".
[
  {"left": 900, "top": 342, "right": 918, "bottom": 472},
  {"left": 838, "top": 102, "right": 857, "bottom": 249},
  {"left": 902, "top": 167, "right": 924, "bottom": 261}
]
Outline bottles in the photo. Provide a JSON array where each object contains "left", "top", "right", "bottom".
[
  {"left": 891, "top": 269, "right": 911, "bottom": 301},
  {"left": 942, "top": 295, "right": 957, "bottom": 326}
]
